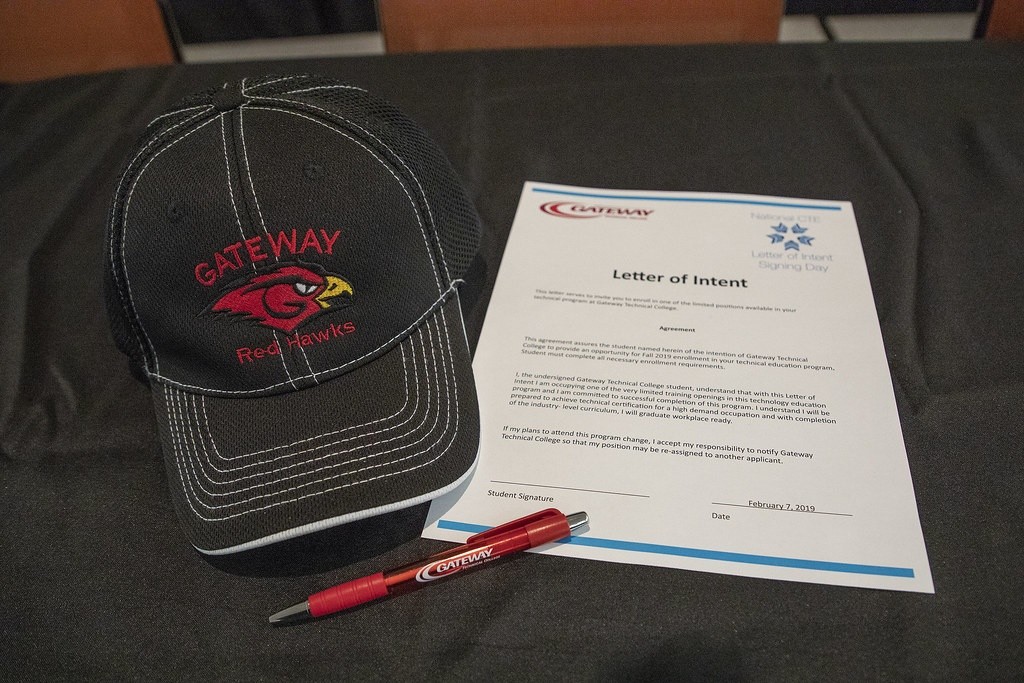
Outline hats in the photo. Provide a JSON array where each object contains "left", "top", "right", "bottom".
[{"left": 102, "top": 73, "right": 482, "bottom": 555}]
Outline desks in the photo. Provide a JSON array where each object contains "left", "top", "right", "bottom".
[{"left": 0, "top": 41, "right": 1024, "bottom": 683}]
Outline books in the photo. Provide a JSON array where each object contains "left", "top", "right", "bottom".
[{"left": 158, "top": 0, "right": 384, "bottom": 66}]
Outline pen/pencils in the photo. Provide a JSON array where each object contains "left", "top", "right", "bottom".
[{"left": 265, "top": 507, "right": 591, "bottom": 626}]
